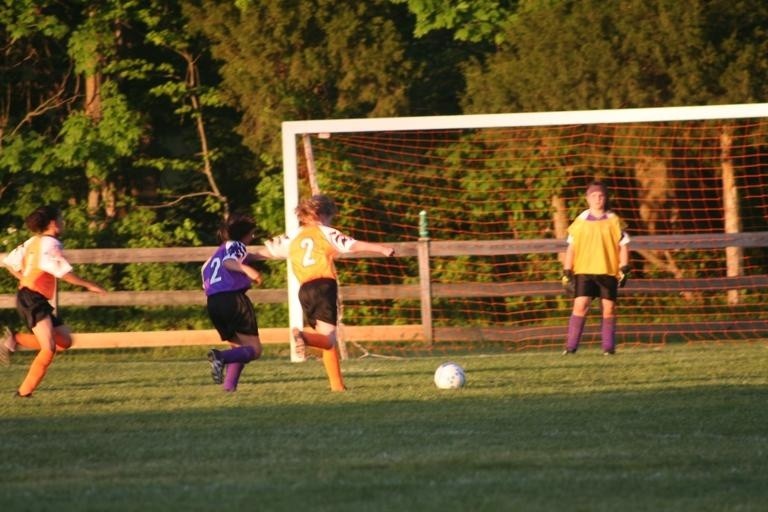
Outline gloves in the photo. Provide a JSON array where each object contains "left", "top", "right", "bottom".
[
  {"left": 562, "top": 269, "right": 575, "bottom": 291},
  {"left": 618, "top": 268, "right": 625, "bottom": 288}
]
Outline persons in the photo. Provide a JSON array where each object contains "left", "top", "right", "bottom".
[
  {"left": 561, "top": 183, "right": 631, "bottom": 356},
  {"left": 258, "top": 194, "right": 395, "bottom": 393},
  {"left": 200, "top": 213, "right": 263, "bottom": 392},
  {"left": 0, "top": 205, "right": 109, "bottom": 399}
]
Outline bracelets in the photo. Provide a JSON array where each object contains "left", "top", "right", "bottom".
[
  {"left": 620, "top": 265, "right": 631, "bottom": 273},
  {"left": 563, "top": 269, "right": 572, "bottom": 276}
]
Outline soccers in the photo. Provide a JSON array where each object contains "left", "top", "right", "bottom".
[{"left": 433, "top": 362, "right": 467, "bottom": 391}]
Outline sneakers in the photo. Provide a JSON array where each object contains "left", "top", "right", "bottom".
[
  {"left": 603, "top": 351, "right": 616, "bottom": 355},
  {"left": 1, "top": 326, "right": 16, "bottom": 367},
  {"left": 12, "top": 392, "right": 32, "bottom": 399},
  {"left": 292, "top": 327, "right": 305, "bottom": 358},
  {"left": 207, "top": 348, "right": 224, "bottom": 384},
  {"left": 562, "top": 350, "right": 576, "bottom": 356}
]
